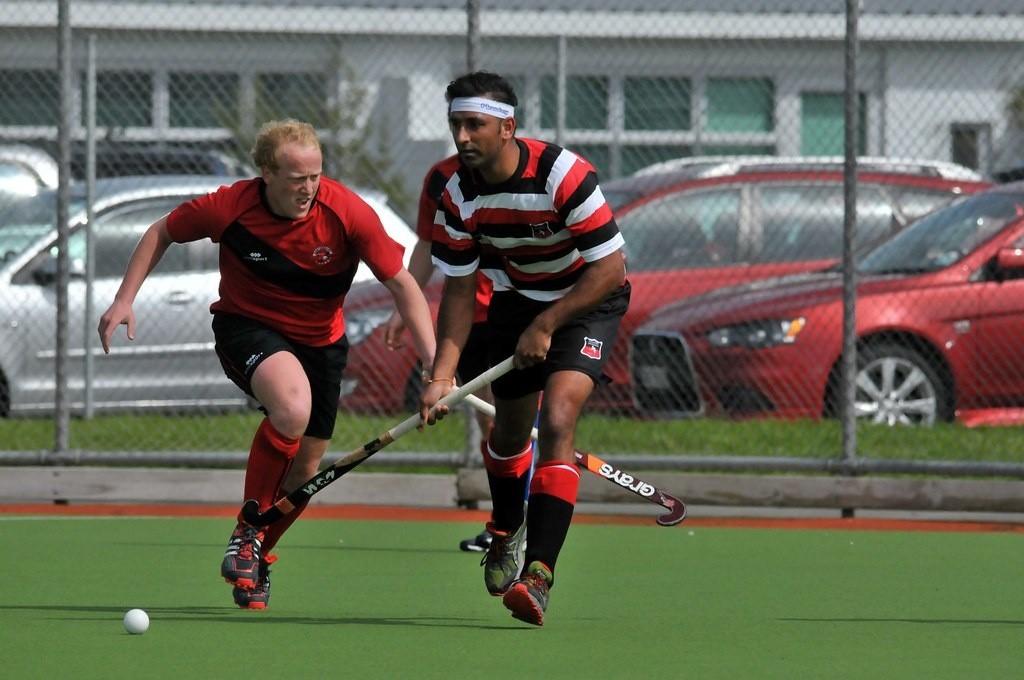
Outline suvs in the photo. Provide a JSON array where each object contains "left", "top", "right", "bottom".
[{"left": 337, "top": 168, "right": 1003, "bottom": 417}]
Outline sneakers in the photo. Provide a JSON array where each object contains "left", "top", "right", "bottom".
[
  {"left": 231, "top": 552, "right": 279, "bottom": 610},
  {"left": 480, "top": 515, "right": 527, "bottom": 596},
  {"left": 504, "top": 561, "right": 554, "bottom": 626},
  {"left": 221, "top": 510, "right": 269, "bottom": 594},
  {"left": 460, "top": 532, "right": 493, "bottom": 552}
]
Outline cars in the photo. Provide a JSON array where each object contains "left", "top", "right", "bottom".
[
  {"left": 620, "top": 176, "right": 1019, "bottom": 437},
  {"left": 4, "top": 123, "right": 257, "bottom": 207},
  {"left": 600, "top": 152, "right": 989, "bottom": 233},
  {"left": 0, "top": 175, "right": 445, "bottom": 425}
]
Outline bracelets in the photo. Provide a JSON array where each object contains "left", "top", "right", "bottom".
[{"left": 426, "top": 377, "right": 455, "bottom": 387}]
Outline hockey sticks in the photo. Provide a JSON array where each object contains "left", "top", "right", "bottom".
[
  {"left": 241, "top": 355, "right": 516, "bottom": 528},
  {"left": 420, "top": 368, "right": 688, "bottom": 527}
]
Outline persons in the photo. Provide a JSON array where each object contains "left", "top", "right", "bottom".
[
  {"left": 97, "top": 117, "right": 458, "bottom": 610},
  {"left": 379, "top": 70, "right": 633, "bottom": 626}
]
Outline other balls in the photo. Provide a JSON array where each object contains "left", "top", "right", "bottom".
[{"left": 123, "top": 609, "right": 150, "bottom": 635}]
